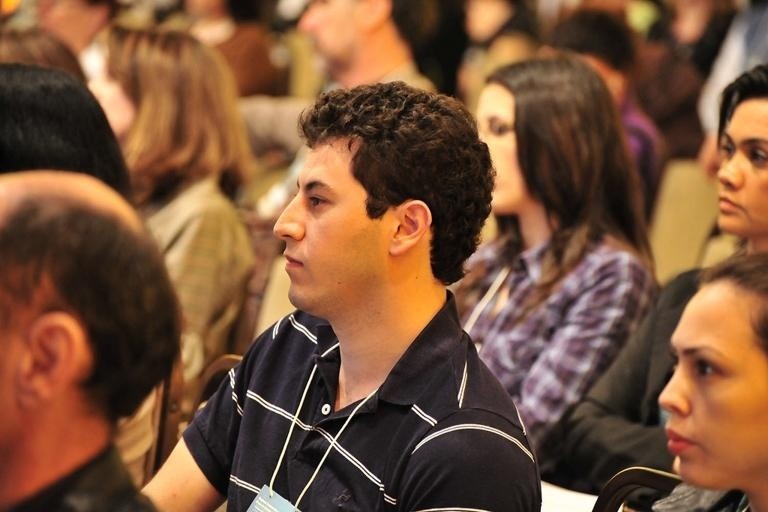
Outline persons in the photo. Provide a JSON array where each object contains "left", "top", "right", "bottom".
[
  {"left": 652, "top": 252, "right": 768, "bottom": 512},
  {"left": 139, "top": 81, "right": 542, "bottom": 512},
  {"left": 1, "top": 170, "right": 182, "bottom": 512},
  {"left": 0, "top": 1, "right": 767, "bottom": 467},
  {"left": 454, "top": 58, "right": 659, "bottom": 472},
  {"left": 559, "top": 64, "right": 768, "bottom": 488}
]
